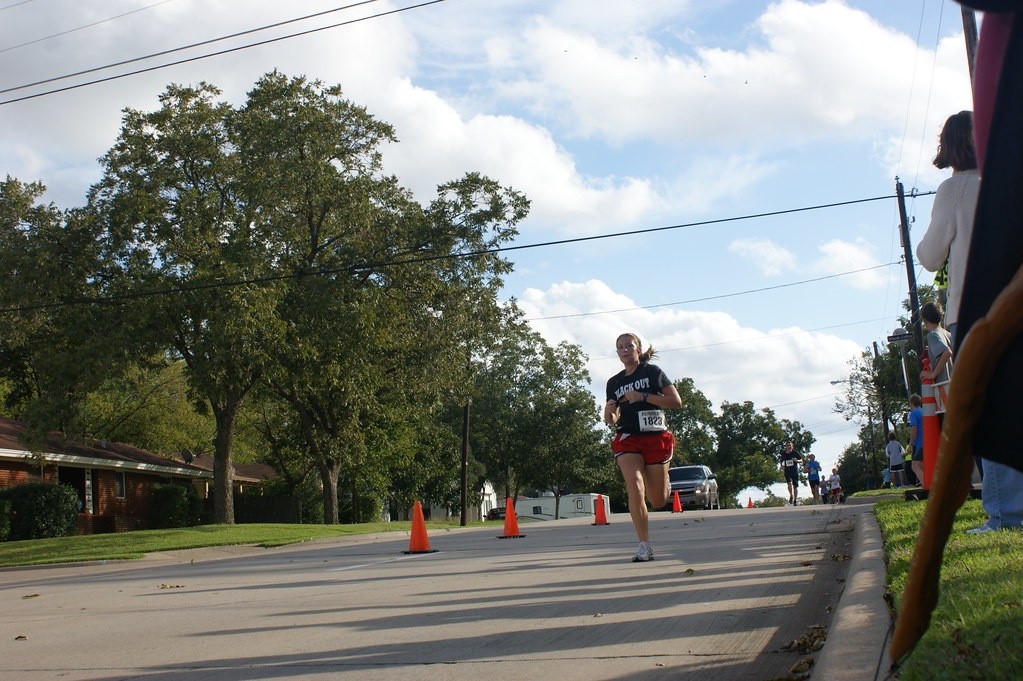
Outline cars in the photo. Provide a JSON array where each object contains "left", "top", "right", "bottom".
[{"left": 483, "top": 508, "right": 519, "bottom": 521}]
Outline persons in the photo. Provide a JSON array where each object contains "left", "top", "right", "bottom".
[
  {"left": 920, "top": 302, "right": 983, "bottom": 489},
  {"left": 604, "top": 333, "right": 682, "bottom": 562},
  {"left": 907, "top": 393, "right": 923, "bottom": 487},
  {"left": 882, "top": 465, "right": 891, "bottom": 488},
  {"left": 780, "top": 441, "right": 803, "bottom": 506},
  {"left": 885, "top": 432, "right": 916, "bottom": 487},
  {"left": 916, "top": 111, "right": 1023, "bottom": 533},
  {"left": 819, "top": 468, "right": 844, "bottom": 504},
  {"left": 803, "top": 454, "right": 822, "bottom": 505}
]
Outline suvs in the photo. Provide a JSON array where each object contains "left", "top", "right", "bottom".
[{"left": 657, "top": 464, "right": 720, "bottom": 510}]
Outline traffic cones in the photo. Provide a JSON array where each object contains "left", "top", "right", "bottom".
[
  {"left": 591, "top": 495, "right": 611, "bottom": 525},
  {"left": 401, "top": 500, "right": 440, "bottom": 553},
  {"left": 496, "top": 497, "right": 527, "bottom": 539},
  {"left": 747, "top": 497, "right": 753, "bottom": 508},
  {"left": 671, "top": 491, "right": 685, "bottom": 513}
]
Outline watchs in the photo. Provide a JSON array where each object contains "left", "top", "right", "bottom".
[
  {"left": 643, "top": 392, "right": 648, "bottom": 402},
  {"left": 909, "top": 443, "right": 913, "bottom": 446}
]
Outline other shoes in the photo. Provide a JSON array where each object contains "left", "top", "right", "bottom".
[
  {"left": 789, "top": 498, "right": 797, "bottom": 506},
  {"left": 965, "top": 525, "right": 995, "bottom": 532}
]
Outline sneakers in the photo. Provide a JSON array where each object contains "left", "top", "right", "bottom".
[{"left": 633, "top": 542, "right": 654, "bottom": 561}]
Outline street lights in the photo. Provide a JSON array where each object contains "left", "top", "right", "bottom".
[{"left": 831, "top": 379, "right": 889, "bottom": 467}]
